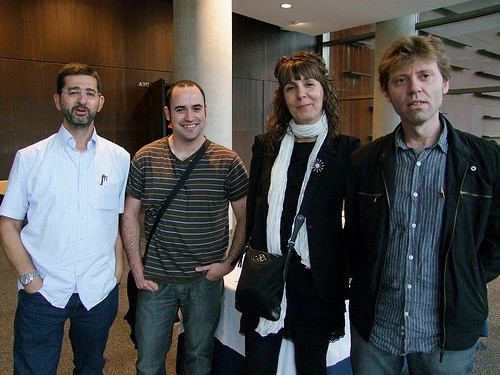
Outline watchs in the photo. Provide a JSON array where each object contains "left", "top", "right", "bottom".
[{"left": 20, "top": 271, "right": 40, "bottom": 286}]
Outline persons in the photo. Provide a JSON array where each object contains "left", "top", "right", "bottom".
[
  {"left": 235, "top": 52, "right": 360, "bottom": 375},
  {"left": 121, "top": 80, "right": 249, "bottom": 375},
  {"left": 0, "top": 64, "right": 131, "bottom": 375},
  {"left": 348, "top": 33, "right": 500, "bottom": 375}
]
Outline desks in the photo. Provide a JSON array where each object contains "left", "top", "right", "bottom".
[{"left": 176, "top": 257, "right": 488, "bottom": 375}]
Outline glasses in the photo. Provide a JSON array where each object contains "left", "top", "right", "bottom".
[{"left": 60, "top": 90, "right": 102, "bottom": 99}]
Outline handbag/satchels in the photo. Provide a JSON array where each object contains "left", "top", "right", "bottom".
[
  {"left": 235, "top": 249, "right": 290, "bottom": 320},
  {"left": 124, "top": 254, "right": 146, "bottom": 347}
]
